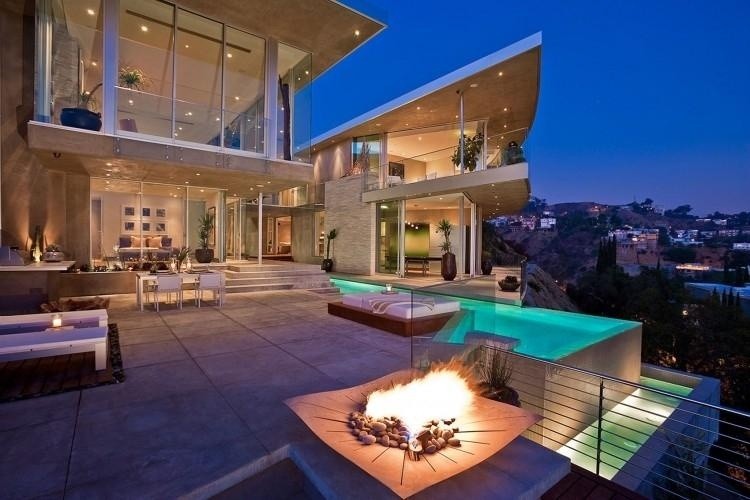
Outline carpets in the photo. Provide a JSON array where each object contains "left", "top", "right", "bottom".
[{"left": 0, "top": 323, "right": 125, "bottom": 404}]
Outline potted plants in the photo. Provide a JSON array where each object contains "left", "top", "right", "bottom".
[
  {"left": 468, "top": 335, "right": 521, "bottom": 408},
  {"left": 60, "top": 64, "right": 154, "bottom": 131},
  {"left": 436, "top": 218, "right": 457, "bottom": 281},
  {"left": 323, "top": 229, "right": 336, "bottom": 272},
  {"left": 499, "top": 275, "right": 521, "bottom": 292},
  {"left": 195, "top": 213, "right": 214, "bottom": 263},
  {"left": 44, "top": 244, "right": 64, "bottom": 262}
]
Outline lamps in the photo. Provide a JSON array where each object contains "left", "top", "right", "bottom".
[
  {"left": 32, "top": 225, "right": 43, "bottom": 262},
  {"left": 47, "top": 313, "right": 64, "bottom": 331}
]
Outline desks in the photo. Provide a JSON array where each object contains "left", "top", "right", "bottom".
[
  {"left": 0, "top": 261, "right": 77, "bottom": 294},
  {"left": 0, "top": 308, "right": 109, "bottom": 371},
  {"left": 119, "top": 247, "right": 159, "bottom": 257},
  {"left": 286, "top": 435, "right": 571, "bottom": 500},
  {"left": 405, "top": 256, "right": 443, "bottom": 276}
]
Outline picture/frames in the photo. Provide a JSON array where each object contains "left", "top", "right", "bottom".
[
  {"left": 121, "top": 205, "right": 168, "bottom": 235},
  {"left": 208, "top": 206, "right": 216, "bottom": 245},
  {"left": 389, "top": 162, "right": 404, "bottom": 180}
]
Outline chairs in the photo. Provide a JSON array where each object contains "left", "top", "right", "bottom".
[
  {"left": 135, "top": 270, "right": 225, "bottom": 313},
  {"left": 389, "top": 171, "right": 436, "bottom": 187}
]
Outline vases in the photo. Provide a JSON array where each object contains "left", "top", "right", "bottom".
[{"left": 481, "top": 262, "right": 492, "bottom": 275}]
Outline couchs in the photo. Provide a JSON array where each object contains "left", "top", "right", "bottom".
[{"left": 119, "top": 236, "right": 173, "bottom": 261}]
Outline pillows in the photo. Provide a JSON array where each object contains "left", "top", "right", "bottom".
[
  {"left": 130, "top": 236, "right": 147, "bottom": 248},
  {"left": 147, "top": 236, "right": 163, "bottom": 247}
]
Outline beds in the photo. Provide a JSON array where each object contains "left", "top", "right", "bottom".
[{"left": 328, "top": 292, "right": 460, "bottom": 337}]
